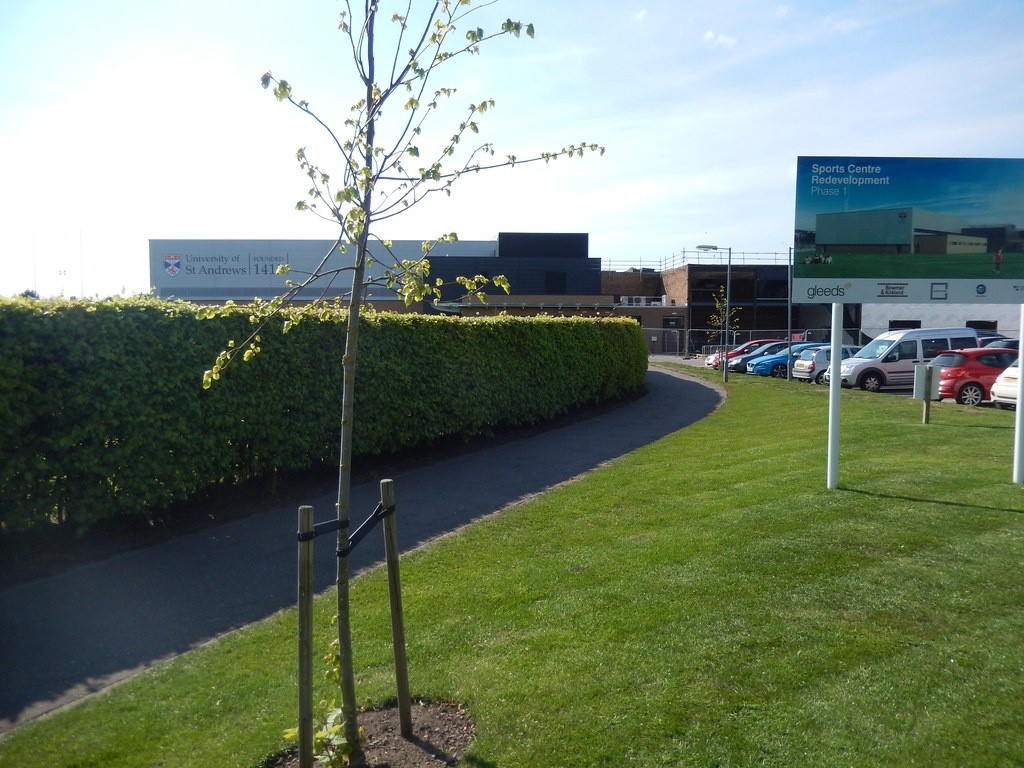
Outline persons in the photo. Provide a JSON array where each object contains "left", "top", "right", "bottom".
[
  {"left": 992, "top": 249, "right": 1003, "bottom": 275},
  {"left": 804, "top": 253, "right": 833, "bottom": 264}
]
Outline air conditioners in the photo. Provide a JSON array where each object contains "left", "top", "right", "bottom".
[
  {"left": 633, "top": 296, "right": 647, "bottom": 307},
  {"left": 651, "top": 301, "right": 662, "bottom": 307}
]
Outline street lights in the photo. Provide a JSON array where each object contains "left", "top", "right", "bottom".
[
  {"left": 695, "top": 244, "right": 732, "bottom": 384},
  {"left": 786, "top": 243, "right": 814, "bottom": 380}
]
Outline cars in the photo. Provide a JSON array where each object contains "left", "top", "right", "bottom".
[
  {"left": 746, "top": 342, "right": 831, "bottom": 378},
  {"left": 712, "top": 338, "right": 787, "bottom": 369},
  {"left": 704, "top": 351, "right": 728, "bottom": 368},
  {"left": 926, "top": 347, "right": 1019, "bottom": 407},
  {"left": 989, "top": 357, "right": 1018, "bottom": 408},
  {"left": 791, "top": 344, "right": 862, "bottom": 384},
  {"left": 973, "top": 329, "right": 1019, "bottom": 349},
  {"left": 727, "top": 341, "right": 814, "bottom": 373}
]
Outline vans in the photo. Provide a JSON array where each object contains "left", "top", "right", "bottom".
[{"left": 823, "top": 327, "right": 981, "bottom": 392}]
